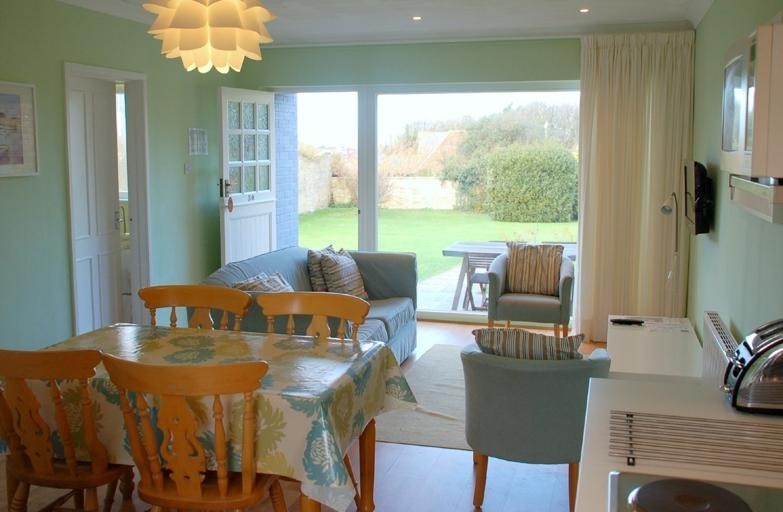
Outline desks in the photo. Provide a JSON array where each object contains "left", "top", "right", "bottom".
[{"left": 443, "top": 240, "right": 577, "bottom": 311}]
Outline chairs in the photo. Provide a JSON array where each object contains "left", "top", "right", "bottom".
[
  {"left": 0, "top": 347, "right": 136, "bottom": 512},
  {"left": 101, "top": 349, "right": 299, "bottom": 511},
  {"left": 138, "top": 286, "right": 247, "bottom": 331},
  {"left": 464, "top": 250, "right": 496, "bottom": 311},
  {"left": 253, "top": 291, "right": 370, "bottom": 342}
]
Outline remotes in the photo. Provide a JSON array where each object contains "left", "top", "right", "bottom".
[{"left": 609, "top": 317, "right": 645, "bottom": 325}]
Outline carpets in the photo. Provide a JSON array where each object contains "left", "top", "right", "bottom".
[{"left": 371, "top": 344, "right": 484, "bottom": 448}]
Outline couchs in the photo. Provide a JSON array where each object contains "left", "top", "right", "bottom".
[
  {"left": 462, "top": 342, "right": 612, "bottom": 511},
  {"left": 486, "top": 249, "right": 575, "bottom": 337},
  {"left": 185, "top": 245, "right": 418, "bottom": 364}
]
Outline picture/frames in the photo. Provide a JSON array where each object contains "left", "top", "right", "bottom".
[{"left": 2, "top": 79, "right": 40, "bottom": 177}]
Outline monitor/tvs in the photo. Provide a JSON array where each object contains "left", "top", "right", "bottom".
[{"left": 680, "top": 158, "right": 714, "bottom": 236}]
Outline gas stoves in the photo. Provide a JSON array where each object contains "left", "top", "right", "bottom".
[{"left": 609, "top": 470, "right": 782, "bottom": 512}]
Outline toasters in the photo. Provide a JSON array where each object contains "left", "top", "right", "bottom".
[{"left": 719, "top": 319, "right": 783, "bottom": 417}]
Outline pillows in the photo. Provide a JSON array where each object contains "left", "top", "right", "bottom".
[
  {"left": 506, "top": 240, "right": 563, "bottom": 299},
  {"left": 233, "top": 271, "right": 269, "bottom": 296},
  {"left": 472, "top": 329, "right": 581, "bottom": 357},
  {"left": 320, "top": 246, "right": 368, "bottom": 302},
  {"left": 310, "top": 244, "right": 335, "bottom": 295},
  {"left": 249, "top": 272, "right": 294, "bottom": 304}
]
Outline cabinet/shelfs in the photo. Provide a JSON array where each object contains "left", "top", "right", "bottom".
[{"left": 606, "top": 314, "right": 701, "bottom": 376}]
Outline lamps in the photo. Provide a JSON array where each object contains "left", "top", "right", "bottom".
[
  {"left": 142, "top": 0, "right": 275, "bottom": 73},
  {"left": 662, "top": 194, "right": 682, "bottom": 317}
]
